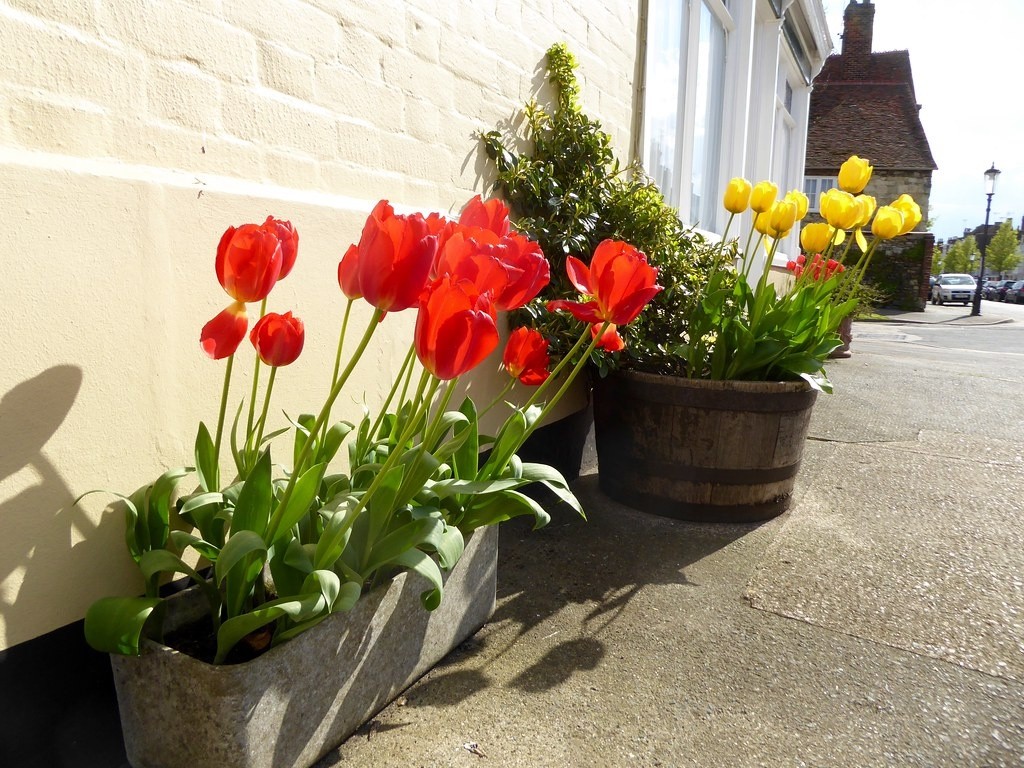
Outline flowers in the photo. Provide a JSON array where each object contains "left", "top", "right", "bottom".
[
  {"left": 473, "top": 40, "right": 922, "bottom": 396},
  {"left": 59, "top": 195, "right": 668, "bottom": 659},
  {"left": 786, "top": 251, "right": 890, "bottom": 319}
]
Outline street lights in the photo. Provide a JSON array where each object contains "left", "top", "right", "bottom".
[
  {"left": 971, "top": 162, "right": 1002, "bottom": 317},
  {"left": 969, "top": 252, "right": 976, "bottom": 274}
]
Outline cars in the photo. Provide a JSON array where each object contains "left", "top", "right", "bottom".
[
  {"left": 932, "top": 272, "right": 978, "bottom": 306},
  {"left": 928, "top": 275, "right": 1024, "bottom": 306}
]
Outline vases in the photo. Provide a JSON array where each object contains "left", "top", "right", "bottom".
[
  {"left": 590, "top": 372, "right": 819, "bottom": 522},
  {"left": 820, "top": 312, "right": 855, "bottom": 360},
  {"left": 108, "top": 520, "right": 499, "bottom": 768}
]
